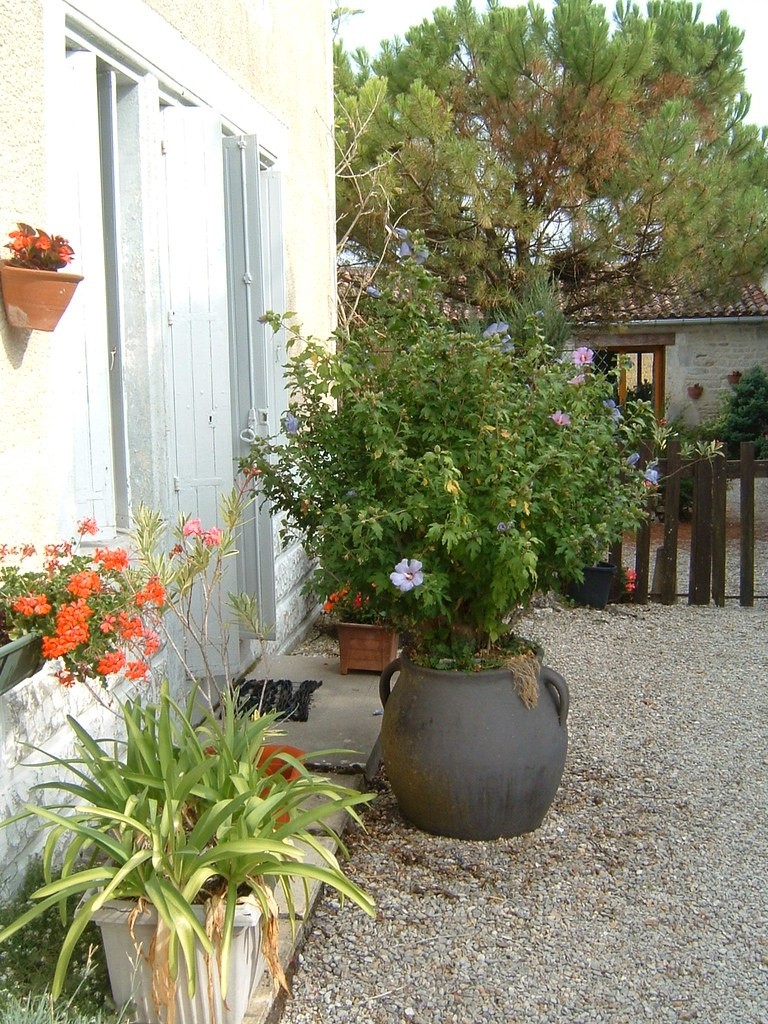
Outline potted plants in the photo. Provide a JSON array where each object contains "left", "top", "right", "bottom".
[
  {"left": 567, "top": 561, "right": 619, "bottom": 611},
  {"left": 0, "top": 718, "right": 382, "bottom": 1024}
]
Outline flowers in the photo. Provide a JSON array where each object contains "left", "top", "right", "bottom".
[
  {"left": 241, "top": 216, "right": 729, "bottom": 668},
  {"left": 621, "top": 566, "right": 636, "bottom": 592},
  {"left": 0, "top": 517, "right": 168, "bottom": 707},
  {"left": 128, "top": 466, "right": 278, "bottom": 756},
  {"left": 4, "top": 220, "right": 75, "bottom": 272}
]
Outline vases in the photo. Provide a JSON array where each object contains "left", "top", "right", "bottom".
[
  {"left": 727, "top": 371, "right": 742, "bottom": 385},
  {"left": 326, "top": 584, "right": 383, "bottom": 625},
  {"left": 338, "top": 624, "right": 399, "bottom": 676},
  {"left": 687, "top": 383, "right": 703, "bottom": 399},
  {"left": 203, "top": 743, "right": 306, "bottom": 826},
  {"left": 0, "top": 631, "right": 46, "bottom": 698},
  {"left": 376, "top": 635, "right": 570, "bottom": 844},
  {"left": 0, "top": 265, "right": 84, "bottom": 332}
]
